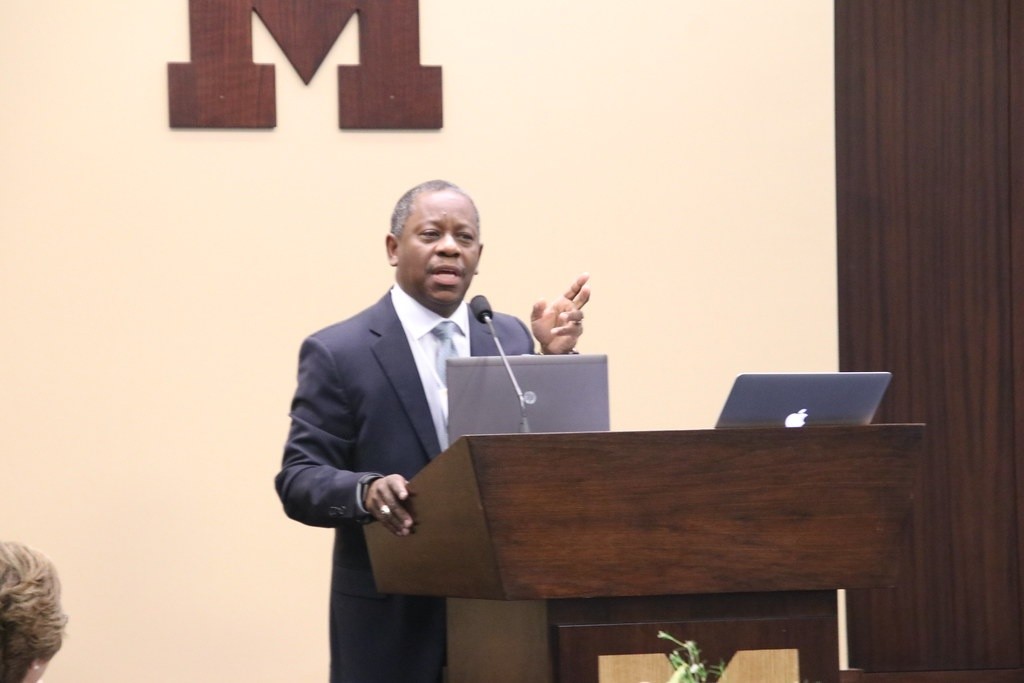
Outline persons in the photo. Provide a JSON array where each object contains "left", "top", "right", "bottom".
[
  {"left": 274, "top": 180, "right": 591, "bottom": 683},
  {"left": 0, "top": 542, "right": 68, "bottom": 683}
]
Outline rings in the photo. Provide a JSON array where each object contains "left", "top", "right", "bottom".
[{"left": 380, "top": 506, "right": 392, "bottom": 517}]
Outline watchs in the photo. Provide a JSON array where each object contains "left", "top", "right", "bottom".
[{"left": 361, "top": 476, "right": 381, "bottom": 506}]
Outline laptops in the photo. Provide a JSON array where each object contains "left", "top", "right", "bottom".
[
  {"left": 447, "top": 355, "right": 611, "bottom": 445},
  {"left": 716, "top": 371, "right": 892, "bottom": 429}
]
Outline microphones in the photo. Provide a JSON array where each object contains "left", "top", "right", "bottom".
[{"left": 471, "top": 294, "right": 530, "bottom": 434}]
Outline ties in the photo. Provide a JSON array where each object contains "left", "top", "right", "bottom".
[{"left": 430, "top": 320, "right": 463, "bottom": 385}]
